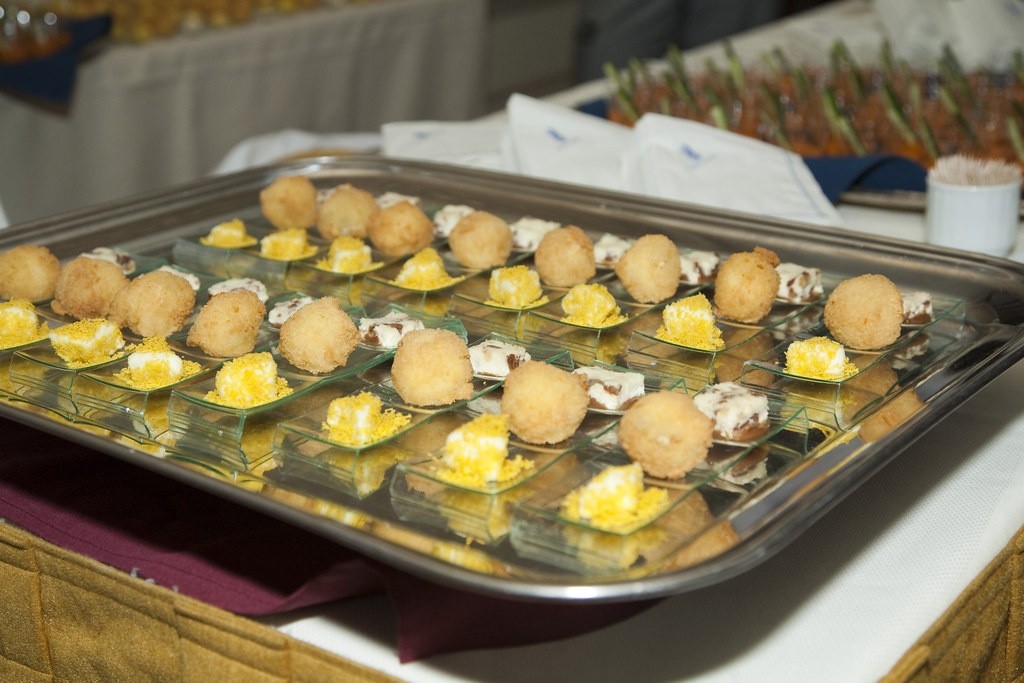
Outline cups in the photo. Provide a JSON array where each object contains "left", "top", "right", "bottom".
[{"left": 926, "top": 179, "right": 1019, "bottom": 258}]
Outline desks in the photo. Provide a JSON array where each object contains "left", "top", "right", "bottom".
[{"left": 0, "top": 349, "right": 1022, "bottom": 681}]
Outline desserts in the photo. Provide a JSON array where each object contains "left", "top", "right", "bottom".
[{"left": 0, "top": 172, "right": 932, "bottom": 533}]
